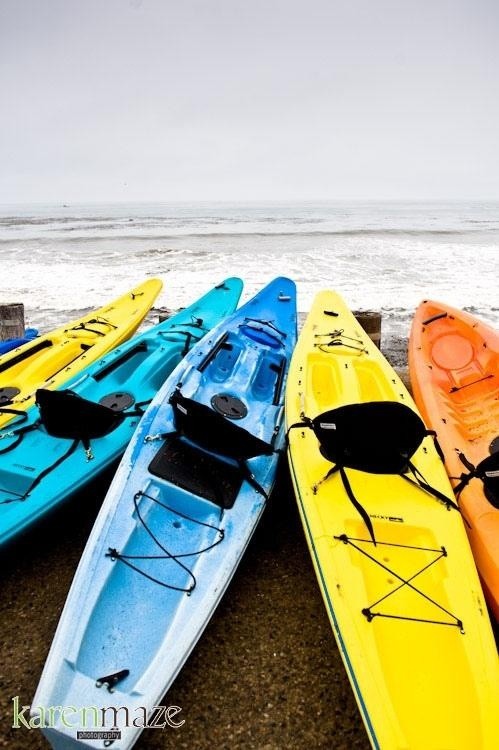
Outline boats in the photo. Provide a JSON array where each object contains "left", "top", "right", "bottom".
[
  {"left": 0, "top": 276, "right": 253, "bottom": 545},
  {"left": 408, "top": 297, "right": 499, "bottom": 616},
  {"left": 28, "top": 275, "right": 306, "bottom": 750},
  {"left": 290, "top": 286, "right": 499, "bottom": 750},
  {"left": 0, "top": 278, "right": 166, "bottom": 429}
]
